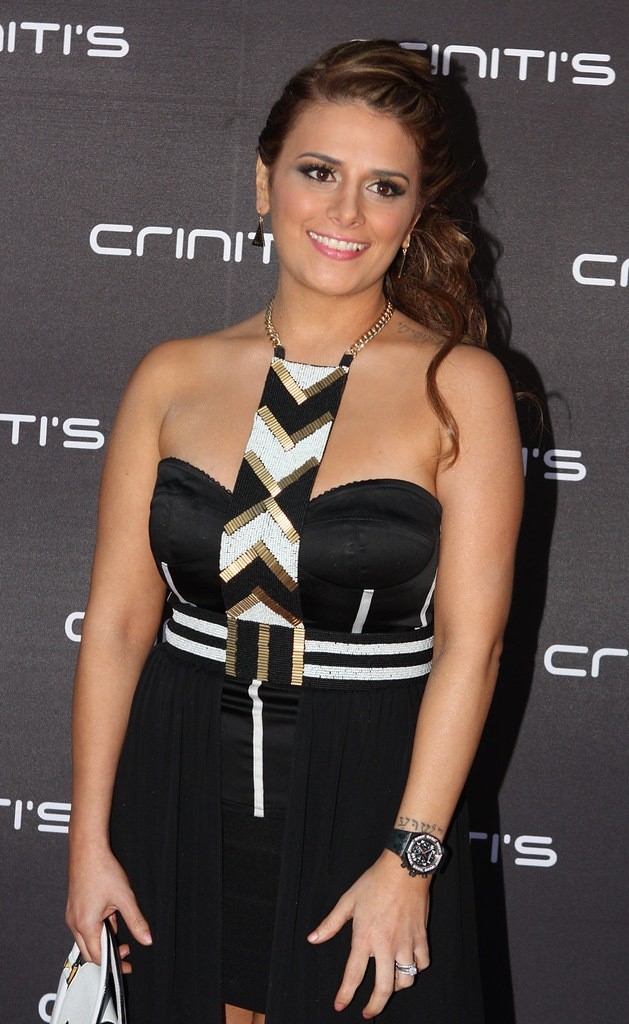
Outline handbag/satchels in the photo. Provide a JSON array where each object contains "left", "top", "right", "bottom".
[{"left": 37, "top": 918, "right": 125, "bottom": 1024}]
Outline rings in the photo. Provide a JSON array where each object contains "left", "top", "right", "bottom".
[{"left": 394, "top": 960, "right": 417, "bottom": 977}]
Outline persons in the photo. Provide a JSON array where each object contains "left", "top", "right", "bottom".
[{"left": 63, "top": 37, "right": 525, "bottom": 1024}]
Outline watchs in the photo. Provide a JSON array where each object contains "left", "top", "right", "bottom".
[{"left": 382, "top": 827, "right": 450, "bottom": 880}]
